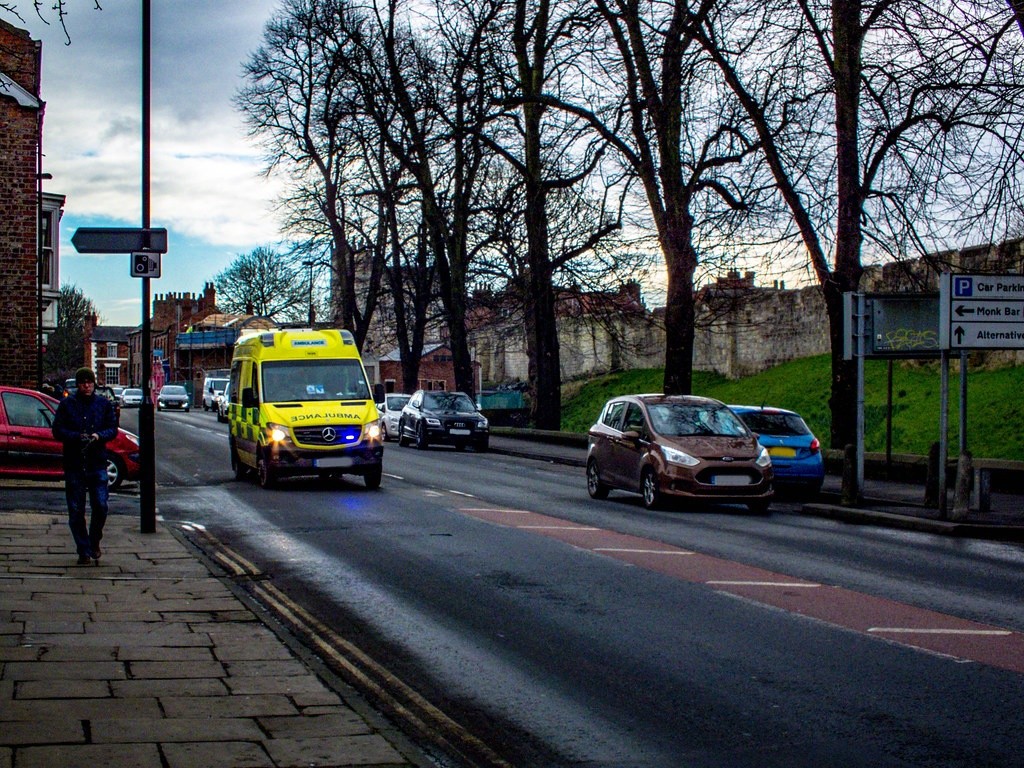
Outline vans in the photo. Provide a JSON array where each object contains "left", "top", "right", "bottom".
[{"left": 201, "top": 377, "right": 230, "bottom": 411}]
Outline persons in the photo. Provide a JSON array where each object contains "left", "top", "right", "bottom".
[
  {"left": 42, "top": 377, "right": 64, "bottom": 400},
  {"left": 52, "top": 367, "right": 118, "bottom": 565}
]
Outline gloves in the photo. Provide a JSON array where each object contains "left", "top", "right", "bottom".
[{"left": 80, "top": 433, "right": 96, "bottom": 453}]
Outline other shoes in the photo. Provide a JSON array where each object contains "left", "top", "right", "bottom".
[
  {"left": 89, "top": 545, "right": 101, "bottom": 559},
  {"left": 77, "top": 553, "right": 90, "bottom": 564}
]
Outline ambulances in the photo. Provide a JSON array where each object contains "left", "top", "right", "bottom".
[{"left": 224, "top": 325, "right": 386, "bottom": 491}]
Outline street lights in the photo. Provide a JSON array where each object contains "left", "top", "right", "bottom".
[{"left": 302, "top": 261, "right": 314, "bottom": 323}]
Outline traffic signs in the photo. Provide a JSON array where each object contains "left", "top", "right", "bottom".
[{"left": 938, "top": 271, "right": 1024, "bottom": 351}]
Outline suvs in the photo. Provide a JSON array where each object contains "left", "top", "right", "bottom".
[{"left": 397, "top": 389, "right": 489, "bottom": 453}]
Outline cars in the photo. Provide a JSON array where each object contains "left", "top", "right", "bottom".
[
  {"left": 0, "top": 386, "right": 140, "bottom": 491},
  {"left": 375, "top": 392, "right": 412, "bottom": 442},
  {"left": 155, "top": 385, "right": 191, "bottom": 412},
  {"left": 216, "top": 381, "right": 230, "bottom": 423},
  {"left": 585, "top": 392, "right": 777, "bottom": 514},
  {"left": 60, "top": 377, "right": 143, "bottom": 408},
  {"left": 696, "top": 403, "right": 825, "bottom": 501}
]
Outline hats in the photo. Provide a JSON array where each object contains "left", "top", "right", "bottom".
[{"left": 75, "top": 368, "right": 95, "bottom": 383}]
